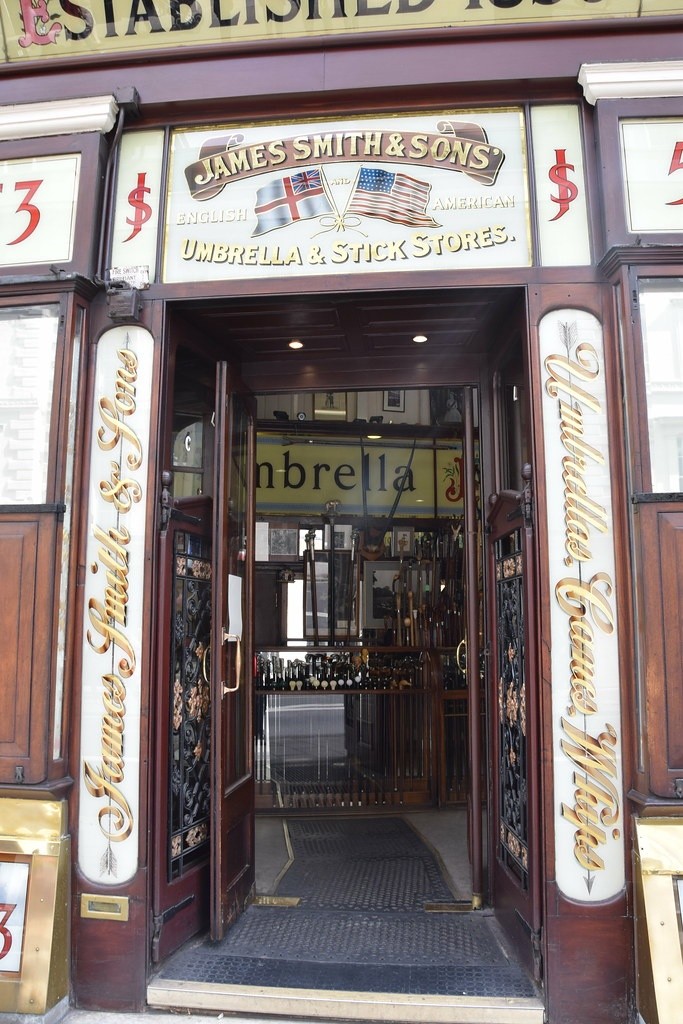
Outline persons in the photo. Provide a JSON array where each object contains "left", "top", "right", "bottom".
[
  {"left": 444, "top": 393, "right": 462, "bottom": 422},
  {"left": 325, "top": 392, "right": 335, "bottom": 409}
]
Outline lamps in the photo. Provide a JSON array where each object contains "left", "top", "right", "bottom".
[
  {"left": 413, "top": 333, "right": 428, "bottom": 343},
  {"left": 288, "top": 339, "right": 304, "bottom": 349}
]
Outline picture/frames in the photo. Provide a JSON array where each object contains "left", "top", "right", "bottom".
[
  {"left": 312, "top": 392, "right": 348, "bottom": 422},
  {"left": 303, "top": 550, "right": 360, "bottom": 638},
  {"left": 364, "top": 560, "right": 433, "bottom": 632},
  {"left": 392, "top": 526, "right": 415, "bottom": 558},
  {"left": 382, "top": 390, "right": 405, "bottom": 412},
  {"left": 268, "top": 521, "right": 299, "bottom": 563},
  {"left": 324, "top": 524, "right": 352, "bottom": 550}
]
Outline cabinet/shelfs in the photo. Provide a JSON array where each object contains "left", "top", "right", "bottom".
[{"left": 252, "top": 645, "right": 484, "bottom": 817}]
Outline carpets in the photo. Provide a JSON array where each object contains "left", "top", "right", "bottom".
[
  {"left": 269, "top": 815, "right": 466, "bottom": 910},
  {"left": 190, "top": 907, "right": 510, "bottom": 968}
]
